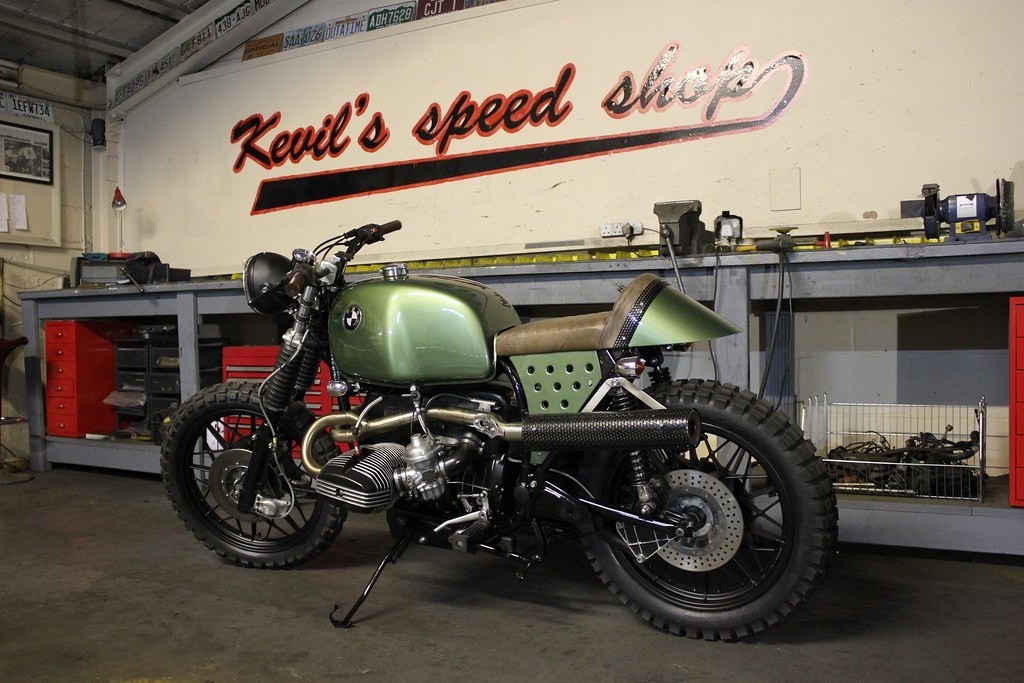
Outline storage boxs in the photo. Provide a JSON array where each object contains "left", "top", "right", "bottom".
[{"left": 43, "top": 318, "right": 223, "bottom": 446}]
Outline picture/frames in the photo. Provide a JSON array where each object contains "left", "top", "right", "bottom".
[{"left": 0, "top": 120, "right": 54, "bottom": 185}]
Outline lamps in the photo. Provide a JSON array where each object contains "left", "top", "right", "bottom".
[{"left": 112, "top": 187, "right": 128, "bottom": 253}]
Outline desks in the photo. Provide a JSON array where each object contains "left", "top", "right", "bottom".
[{"left": 17, "top": 236, "right": 1024, "bottom": 558}]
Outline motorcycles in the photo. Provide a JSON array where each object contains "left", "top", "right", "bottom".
[{"left": 160, "top": 218, "right": 839, "bottom": 642}]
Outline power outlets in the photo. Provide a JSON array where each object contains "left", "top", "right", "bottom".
[{"left": 601, "top": 222, "right": 644, "bottom": 238}]
